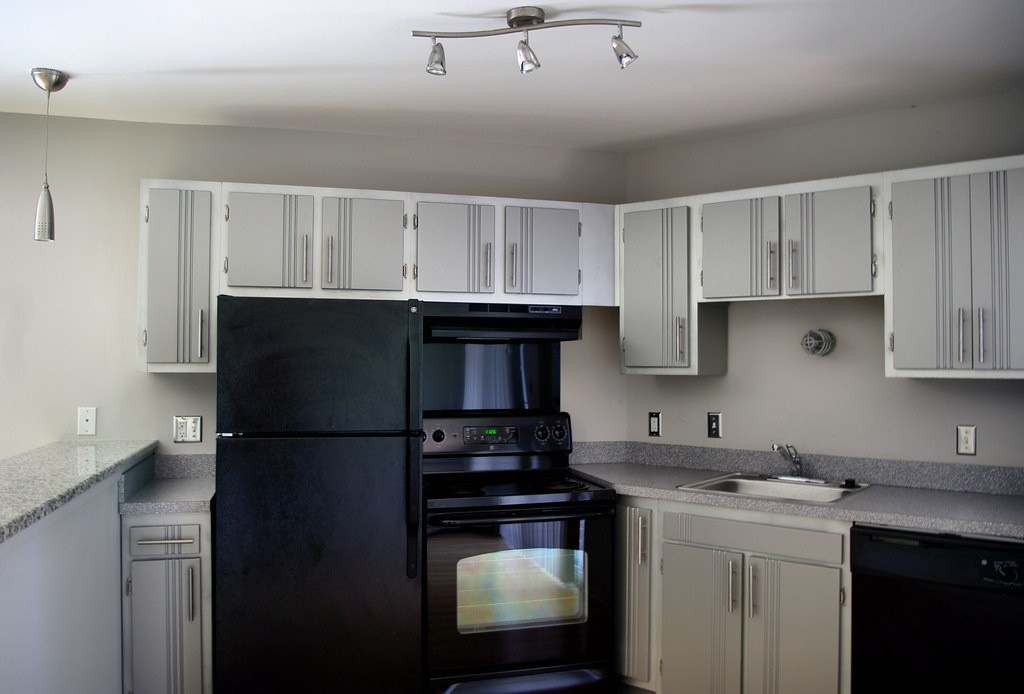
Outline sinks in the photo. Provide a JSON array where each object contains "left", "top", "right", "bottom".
[{"left": 676, "top": 471, "right": 873, "bottom": 504}]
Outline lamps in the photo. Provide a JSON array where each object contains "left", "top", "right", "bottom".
[
  {"left": 30, "top": 68, "right": 69, "bottom": 242},
  {"left": 410, "top": 7, "right": 643, "bottom": 76}
]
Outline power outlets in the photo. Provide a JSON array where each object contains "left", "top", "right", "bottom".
[
  {"left": 173, "top": 416, "right": 202, "bottom": 443},
  {"left": 647, "top": 412, "right": 662, "bottom": 437}
]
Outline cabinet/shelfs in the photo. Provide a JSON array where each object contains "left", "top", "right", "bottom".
[
  {"left": 883, "top": 155, "right": 1024, "bottom": 379},
  {"left": 697, "top": 172, "right": 884, "bottom": 303},
  {"left": 614, "top": 493, "right": 852, "bottom": 694},
  {"left": 615, "top": 195, "right": 728, "bottom": 376},
  {"left": 135, "top": 178, "right": 221, "bottom": 373},
  {"left": 218, "top": 182, "right": 411, "bottom": 301},
  {"left": 122, "top": 511, "right": 212, "bottom": 694},
  {"left": 411, "top": 192, "right": 616, "bottom": 306}
]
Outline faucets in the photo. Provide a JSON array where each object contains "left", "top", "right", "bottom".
[{"left": 771, "top": 442, "right": 801, "bottom": 477}]
